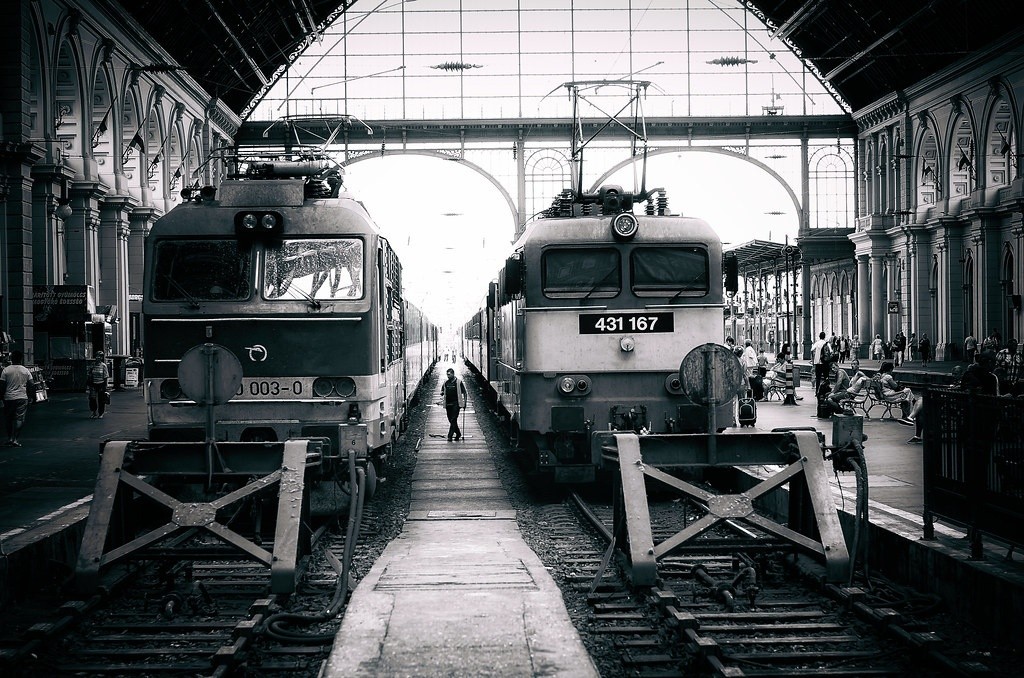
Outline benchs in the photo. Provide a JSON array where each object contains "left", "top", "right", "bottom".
[
  {"left": 826, "top": 376, "right": 911, "bottom": 422},
  {"left": 762, "top": 376, "right": 786, "bottom": 402}
]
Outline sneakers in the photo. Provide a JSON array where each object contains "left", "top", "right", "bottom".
[
  {"left": 897, "top": 417, "right": 914, "bottom": 426},
  {"left": 759, "top": 396, "right": 768, "bottom": 402},
  {"left": 906, "top": 436, "right": 922, "bottom": 444}
]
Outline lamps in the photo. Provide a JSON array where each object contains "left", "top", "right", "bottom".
[{"left": 47, "top": 194, "right": 72, "bottom": 235}]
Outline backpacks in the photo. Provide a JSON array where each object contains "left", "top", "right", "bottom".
[{"left": 820, "top": 342, "right": 830, "bottom": 364}]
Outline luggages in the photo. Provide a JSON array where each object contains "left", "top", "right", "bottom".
[
  {"left": 738, "top": 388, "right": 757, "bottom": 428},
  {"left": 818, "top": 395, "right": 833, "bottom": 418}
]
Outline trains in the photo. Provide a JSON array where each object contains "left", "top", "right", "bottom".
[
  {"left": 454, "top": 184, "right": 744, "bottom": 499},
  {"left": 137, "top": 180, "right": 445, "bottom": 522}
]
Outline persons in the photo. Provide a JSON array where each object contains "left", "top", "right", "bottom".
[
  {"left": 443, "top": 345, "right": 450, "bottom": 362},
  {"left": 872, "top": 334, "right": 884, "bottom": 362},
  {"left": 810, "top": 332, "right": 833, "bottom": 396},
  {"left": 826, "top": 359, "right": 867, "bottom": 407},
  {"left": 964, "top": 332, "right": 977, "bottom": 364},
  {"left": 451, "top": 346, "right": 457, "bottom": 364},
  {"left": 919, "top": 333, "right": 932, "bottom": 366},
  {"left": 759, "top": 353, "right": 804, "bottom": 402},
  {"left": 898, "top": 397, "right": 923, "bottom": 444},
  {"left": 983, "top": 331, "right": 998, "bottom": 352},
  {"left": 952, "top": 339, "right": 1024, "bottom": 396},
  {"left": 893, "top": 332, "right": 906, "bottom": 368},
  {"left": 440, "top": 368, "right": 467, "bottom": 440},
  {"left": 909, "top": 333, "right": 918, "bottom": 363},
  {"left": 874, "top": 362, "right": 916, "bottom": 418},
  {"left": 88, "top": 351, "right": 109, "bottom": 419},
  {"left": 0, "top": 350, "right": 35, "bottom": 446},
  {"left": 827, "top": 332, "right": 860, "bottom": 364},
  {"left": 724, "top": 336, "right": 768, "bottom": 373}
]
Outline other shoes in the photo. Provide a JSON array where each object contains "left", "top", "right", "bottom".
[
  {"left": 3, "top": 440, "right": 13, "bottom": 447},
  {"left": 447, "top": 438, "right": 452, "bottom": 443},
  {"left": 90, "top": 412, "right": 97, "bottom": 419},
  {"left": 97, "top": 413, "right": 104, "bottom": 418},
  {"left": 796, "top": 397, "right": 804, "bottom": 401},
  {"left": 13, "top": 440, "right": 22, "bottom": 447},
  {"left": 454, "top": 433, "right": 461, "bottom": 441}
]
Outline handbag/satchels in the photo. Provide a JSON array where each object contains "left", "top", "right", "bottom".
[
  {"left": 104, "top": 392, "right": 111, "bottom": 405},
  {"left": 87, "top": 367, "right": 94, "bottom": 386}
]
